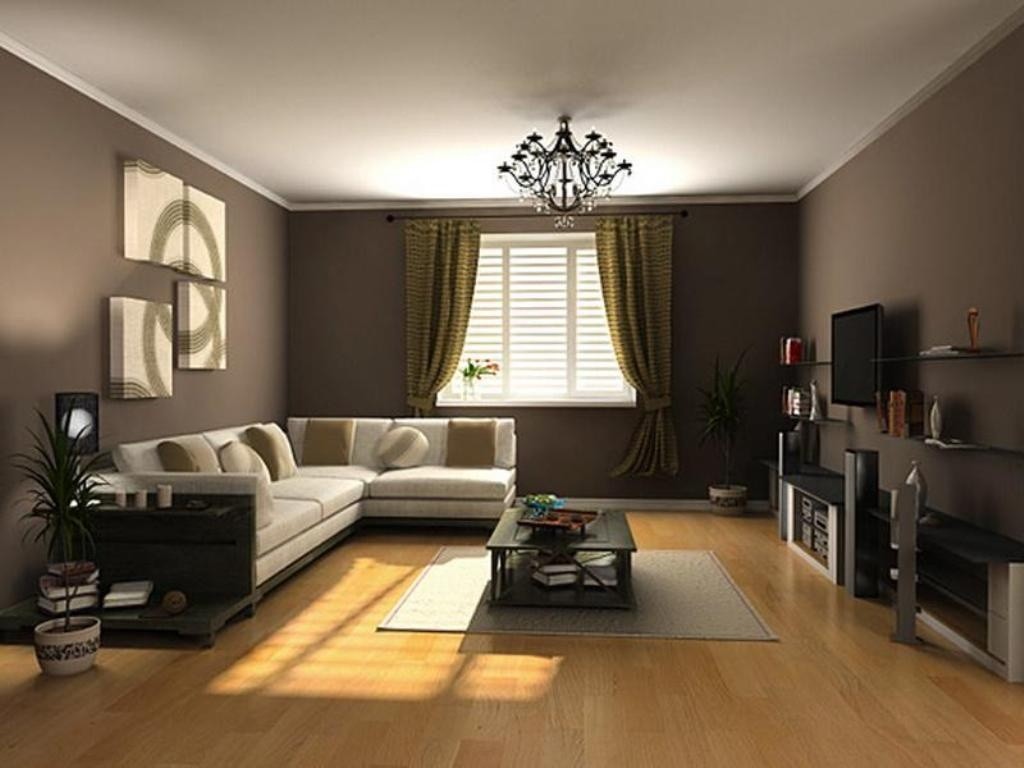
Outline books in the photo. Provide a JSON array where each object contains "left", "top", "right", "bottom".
[
  {"left": 531, "top": 549, "right": 618, "bottom": 588},
  {"left": 38, "top": 561, "right": 153, "bottom": 615}
]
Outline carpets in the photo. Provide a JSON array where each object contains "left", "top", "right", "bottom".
[{"left": 378, "top": 546, "right": 781, "bottom": 642}]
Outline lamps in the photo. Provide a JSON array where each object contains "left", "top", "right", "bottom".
[
  {"left": 56, "top": 392, "right": 99, "bottom": 476},
  {"left": 499, "top": 115, "right": 632, "bottom": 231}
]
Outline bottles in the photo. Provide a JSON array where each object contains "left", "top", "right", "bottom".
[
  {"left": 906, "top": 461, "right": 927, "bottom": 522},
  {"left": 783, "top": 385, "right": 810, "bottom": 416},
  {"left": 781, "top": 337, "right": 803, "bottom": 365},
  {"left": 929, "top": 394, "right": 945, "bottom": 441}
]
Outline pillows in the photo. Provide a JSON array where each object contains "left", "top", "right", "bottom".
[
  {"left": 219, "top": 442, "right": 270, "bottom": 483},
  {"left": 302, "top": 420, "right": 359, "bottom": 465},
  {"left": 446, "top": 421, "right": 496, "bottom": 467},
  {"left": 156, "top": 441, "right": 218, "bottom": 472},
  {"left": 246, "top": 423, "right": 297, "bottom": 480},
  {"left": 377, "top": 427, "right": 430, "bottom": 468}
]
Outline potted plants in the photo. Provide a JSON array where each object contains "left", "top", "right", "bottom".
[
  {"left": 694, "top": 345, "right": 750, "bottom": 516},
  {"left": 9, "top": 396, "right": 111, "bottom": 676}
]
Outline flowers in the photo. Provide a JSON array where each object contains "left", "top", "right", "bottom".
[{"left": 462, "top": 359, "right": 499, "bottom": 379}]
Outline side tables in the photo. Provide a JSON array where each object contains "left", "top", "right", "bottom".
[{"left": 27, "top": 504, "right": 257, "bottom": 646}]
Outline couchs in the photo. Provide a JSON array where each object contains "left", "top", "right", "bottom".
[{"left": 112, "top": 418, "right": 517, "bottom": 604}]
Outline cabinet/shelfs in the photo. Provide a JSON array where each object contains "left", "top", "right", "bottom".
[
  {"left": 884, "top": 353, "right": 1024, "bottom": 460},
  {"left": 761, "top": 362, "right": 844, "bottom": 584},
  {"left": 871, "top": 508, "right": 1024, "bottom": 683}
]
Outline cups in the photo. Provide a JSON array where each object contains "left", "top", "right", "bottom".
[
  {"left": 135, "top": 489, "right": 147, "bottom": 509},
  {"left": 116, "top": 489, "right": 126, "bottom": 507},
  {"left": 156, "top": 484, "right": 172, "bottom": 507}
]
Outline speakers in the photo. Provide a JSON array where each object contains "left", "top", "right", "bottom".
[
  {"left": 845, "top": 447, "right": 879, "bottom": 597},
  {"left": 779, "top": 430, "right": 800, "bottom": 537},
  {"left": 55, "top": 391, "right": 99, "bottom": 455}
]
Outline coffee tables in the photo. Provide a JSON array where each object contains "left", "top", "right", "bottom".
[{"left": 486, "top": 508, "right": 637, "bottom": 613}]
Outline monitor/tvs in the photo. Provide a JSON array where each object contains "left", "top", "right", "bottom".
[{"left": 830, "top": 302, "right": 884, "bottom": 408}]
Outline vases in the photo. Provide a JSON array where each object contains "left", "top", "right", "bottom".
[{"left": 462, "top": 379, "right": 480, "bottom": 403}]
